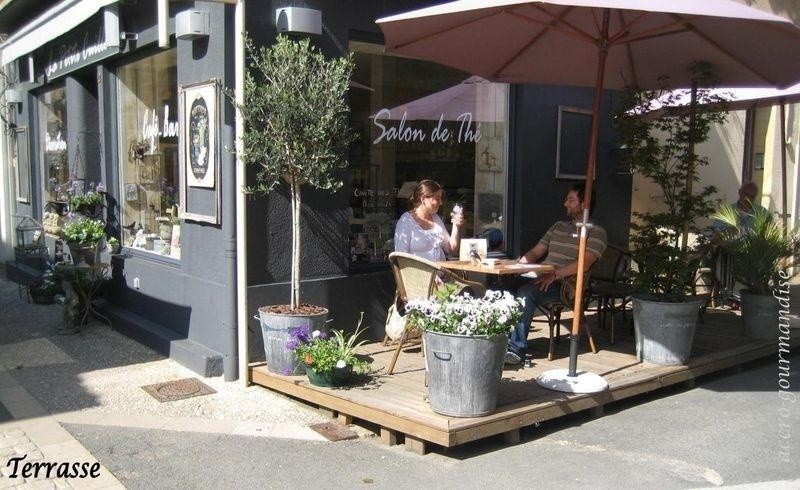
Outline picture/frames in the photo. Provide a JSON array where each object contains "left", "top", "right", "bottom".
[
  {"left": 555, "top": 105, "right": 596, "bottom": 180},
  {"left": 178, "top": 78, "right": 222, "bottom": 226},
  {"left": 13, "top": 125, "right": 31, "bottom": 203}
]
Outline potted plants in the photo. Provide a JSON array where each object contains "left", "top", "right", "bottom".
[
  {"left": 61, "top": 218, "right": 121, "bottom": 268},
  {"left": 215, "top": 31, "right": 361, "bottom": 378},
  {"left": 609, "top": 56, "right": 800, "bottom": 366}
]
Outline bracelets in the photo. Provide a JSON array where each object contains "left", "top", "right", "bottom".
[{"left": 552, "top": 270, "right": 557, "bottom": 279}]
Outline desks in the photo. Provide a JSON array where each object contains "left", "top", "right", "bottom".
[{"left": 437, "top": 258, "right": 554, "bottom": 291}]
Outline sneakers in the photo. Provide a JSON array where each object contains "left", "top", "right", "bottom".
[{"left": 505, "top": 352, "right": 524, "bottom": 365}]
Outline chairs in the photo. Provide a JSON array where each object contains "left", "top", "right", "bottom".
[
  {"left": 383, "top": 251, "right": 487, "bottom": 375},
  {"left": 527, "top": 244, "right": 646, "bottom": 359}
]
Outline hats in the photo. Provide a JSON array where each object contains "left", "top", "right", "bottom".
[{"left": 742, "top": 182, "right": 759, "bottom": 195}]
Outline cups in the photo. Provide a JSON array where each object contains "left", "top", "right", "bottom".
[
  {"left": 470, "top": 243, "right": 479, "bottom": 267},
  {"left": 451, "top": 204, "right": 461, "bottom": 224}
]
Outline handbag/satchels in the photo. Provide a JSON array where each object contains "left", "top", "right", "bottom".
[{"left": 386, "top": 303, "right": 422, "bottom": 341}]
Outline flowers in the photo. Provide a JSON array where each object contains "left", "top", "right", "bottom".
[
  {"left": 41, "top": 259, "right": 56, "bottom": 292},
  {"left": 404, "top": 288, "right": 526, "bottom": 336},
  {"left": 50, "top": 178, "right": 106, "bottom": 204},
  {"left": 286, "top": 310, "right": 371, "bottom": 370}
]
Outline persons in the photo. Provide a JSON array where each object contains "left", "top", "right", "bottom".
[
  {"left": 710, "top": 181, "right": 781, "bottom": 308},
  {"left": 392, "top": 176, "right": 464, "bottom": 266},
  {"left": 501, "top": 182, "right": 610, "bottom": 368}
]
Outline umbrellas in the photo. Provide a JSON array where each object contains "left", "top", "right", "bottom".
[
  {"left": 368, "top": 75, "right": 508, "bottom": 128},
  {"left": 369, "top": 0, "right": 799, "bottom": 396},
  {"left": 614, "top": 83, "right": 799, "bottom": 242}
]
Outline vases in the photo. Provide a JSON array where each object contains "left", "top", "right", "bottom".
[
  {"left": 306, "top": 368, "right": 351, "bottom": 387},
  {"left": 422, "top": 329, "right": 508, "bottom": 418}
]
